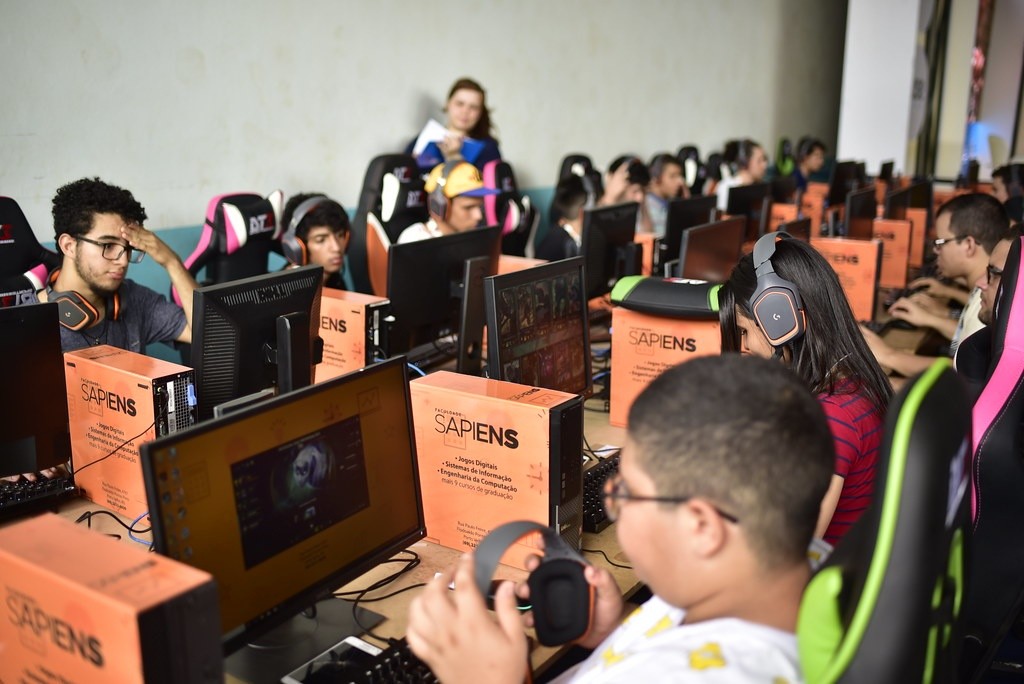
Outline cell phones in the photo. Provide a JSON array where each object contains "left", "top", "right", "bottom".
[{"left": 281, "top": 636, "right": 383, "bottom": 684}]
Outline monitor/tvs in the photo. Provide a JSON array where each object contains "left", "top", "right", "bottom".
[{"left": 0, "top": 158, "right": 980, "bottom": 684}]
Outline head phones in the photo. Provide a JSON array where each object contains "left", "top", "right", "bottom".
[
  {"left": 798, "top": 139, "right": 818, "bottom": 162},
  {"left": 43, "top": 264, "right": 123, "bottom": 330},
  {"left": 282, "top": 196, "right": 352, "bottom": 268},
  {"left": 648, "top": 155, "right": 668, "bottom": 186},
  {"left": 471, "top": 519, "right": 596, "bottom": 684},
  {"left": 737, "top": 140, "right": 747, "bottom": 170},
  {"left": 753, "top": 230, "right": 808, "bottom": 350},
  {"left": 429, "top": 159, "right": 474, "bottom": 220}
]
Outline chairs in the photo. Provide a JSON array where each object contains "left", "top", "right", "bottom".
[
  {"left": 482, "top": 160, "right": 541, "bottom": 258},
  {"left": 347, "top": 153, "right": 430, "bottom": 298},
  {"left": 0, "top": 195, "right": 60, "bottom": 308},
  {"left": 964, "top": 233, "right": 1024, "bottom": 684},
  {"left": 678, "top": 147, "right": 709, "bottom": 195},
  {"left": 790, "top": 355, "right": 974, "bottom": 684},
  {"left": 771, "top": 137, "right": 795, "bottom": 178},
  {"left": 559, "top": 153, "right": 604, "bottom": 207},
  {"left": 168, "top": 190, "right": 285, "bottom": 365}
]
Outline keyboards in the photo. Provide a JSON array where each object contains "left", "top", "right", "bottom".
[
  {"left": 883, "top": 257, "right": 953, "bottom": 310},
  {"left": 863, "top": 320, "right": 890, "bottom": 336},
  {"left": 332, "top": 631, "right": 533, "bottom": 684},
  {"left": 0, "top": 469, "right": 77, "bottom": 527},
  {"left": 580, "top": 449, "right": 624, "bottom": 533}
]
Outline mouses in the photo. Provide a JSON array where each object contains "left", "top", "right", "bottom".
[{"left": 889, "top": 316, "right": 917, "bottom": 332}]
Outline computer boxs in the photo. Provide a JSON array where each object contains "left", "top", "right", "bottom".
[
  {"left": 588, "top": 172, "right": 996, "bottom": 428},
  {"left": 316, "top": 284, "right": 393, "bottom": 384},
  {"left": 408, "top": 369, "right": 584, "bottom": 572},
  {"left": 468, "top": 256, "right": 550, "bottom": 363},
  {"left": 0, "top": 511, "right": 226, "bottom": 684},
  {"left": 61, "top": 344, "right": 199, "bottom": 527}
]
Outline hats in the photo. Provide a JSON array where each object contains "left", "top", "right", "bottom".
[{"left": 424, "top": 160, "right": 500, "bottom": 197}]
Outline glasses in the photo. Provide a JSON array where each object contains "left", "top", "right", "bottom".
[
  {"left": 934, "top": 235, "right": 981, "bottom": 250},
  {"left": 73, "top": 235, "right": 145, "bottom": 264},
  {"left": 604, "top": 474, "right": 740, "bottom": 525},
  {"left": 987, "top": 265, "right": 1003, "bottom": 284}
]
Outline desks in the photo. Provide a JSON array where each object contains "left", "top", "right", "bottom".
[{"left": 63, "top": 265, "right": 960, "bottom": 684}]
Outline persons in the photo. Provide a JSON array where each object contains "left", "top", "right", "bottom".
[
  {"left": 407, "top": 78, "right": 503, "bottom": 172},
  {"left": 278, "top": 193, "right": 351, "bottom": 291},
  {"left": 717, "top": 240, "right": 896, "bottom": 569},
  {"left": 595, "top": 159, "right": 654, "bottom": 237},
  {"left": 648, "top": 152, "right": 691, "bottom": 235},
  {"left": 853, "top": 163, "right": 1024, "bottom": 390},
  {"left": 18, "top": 177, "right": 204, "bottom": 356},
  {"left": 537, "top": 171, "right": 595, "bottom": 263},
  {"left": 714, "top": 141, "right": 768, "bottom": 210},
  {"left": 407, "top": 352, "right": 838, "bottom": 683},
  {"left": 785, "top": 138, "right": 828, "bottom": 196},
  {"left": 396, "top": 161, "right": 494, "bottom": 245}
]
